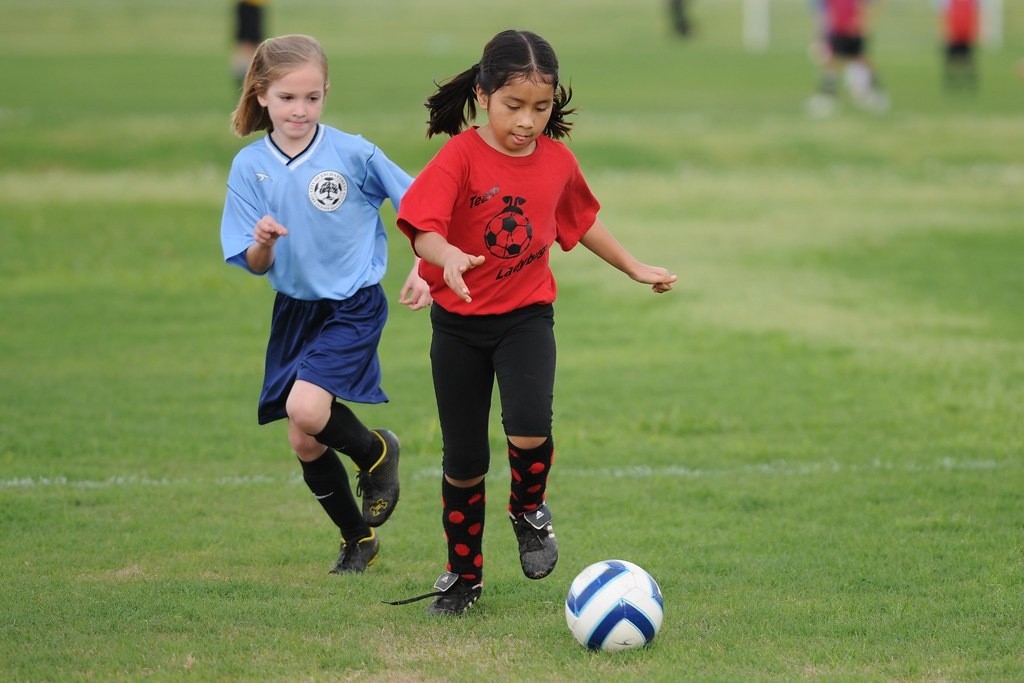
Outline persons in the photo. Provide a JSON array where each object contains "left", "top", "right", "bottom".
[
  {"left": 395, "top": 29, "right": 679, "bottom": 620},
  {"left": 219, "top": 34, "right": 435, "bottom": 576}
]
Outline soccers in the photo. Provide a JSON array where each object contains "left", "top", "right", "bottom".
[{"left": 563, "top": 557, "right": 665, "bottom": 653}]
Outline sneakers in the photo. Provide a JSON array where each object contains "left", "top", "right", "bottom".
[
  {"left": 508, "top": 501, "right": 559, "bottom": 579},
  {"left": 354, "top": 430, "right": 401, "bottom": 525},
  {"left": 328, "top": 527, "right": 380, "bottom": 576},
  {"left": 382, "top": 570, "right": 482, "bottom": 621}
]
